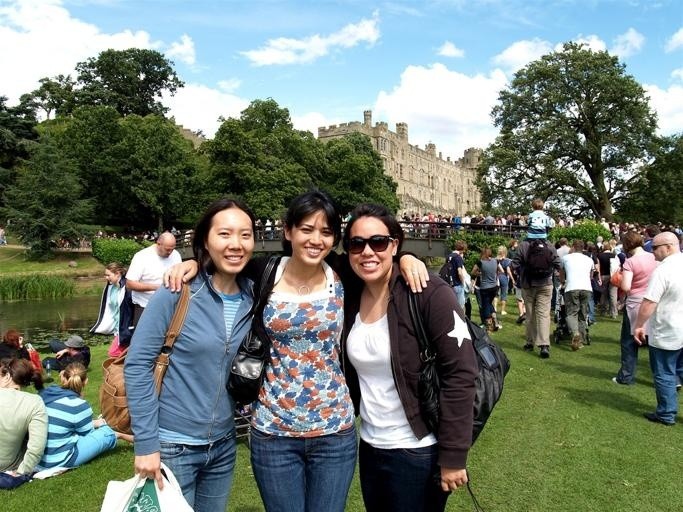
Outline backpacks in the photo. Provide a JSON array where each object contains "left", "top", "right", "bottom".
[
  {"left": 522, "top": 238, "right": 554, "bottom": 280},
  {"left": 439, "top": 255, "right": 459, "bottom": 287}
]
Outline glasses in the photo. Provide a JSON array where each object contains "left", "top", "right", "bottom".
[
  {"left": 649, "top": 244, "right": 667, "bottom": 250},
  {"left": 345, "top": 234, "right": 395, "bottom": 254}
]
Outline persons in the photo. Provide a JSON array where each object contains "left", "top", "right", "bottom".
[
  {"left": 557, "top": 216, "right": 565, "bottom": 229},
  {"left": 399, "top": 206, "right": 574, "bottom": 238},
  {"left": 340, "top": 211, "right": 478, "bottom": 512},
  {"left": 555, "top": 237, "right": 572, "bottom": 269},
  {"left": 561, "top": 240, "right": 594, "bottom": 351},
  {"left": 90, "top": 263, "right": 134, "bottom": 358},
  {"left": 525, "top": 199, "right": 556, "bottom": 240},
  {"left": 597, "top": 244, "right": 621, "bottom": 319},
  {"left": 124, "top": 197, "right": 257, "bottom": 512},
  {"left": 612, "top": 232, "right": 682, "bottom": 392},
  {"left": 635, "top": 232, "right": 683, "bottom": 424},
  {"left": 162, "top": 192, "right": 430, "bottom": 512},
  {"left": 507, "top": 241, "right": 527, "bottom": 324},
  {"left": 551, "top": 241, "right": 561, "bottom": 313},
  {"left": 459, "top": 252, "right": 475, "bottom": 320},
  {"left": 446, "top": 242, "right": 466, "bottom": 315},
  {"left": 0, "top": 330, "right": 31, "bottom": 360},
  {"left": 42, "top": 335, "right": 91, "bottom": 371},
  {"left": 339, "top": 213, "right": 354, "bottom": 233},
  {"left": 254, "top": 217, "right": 286, "bottom": 240},
  {"left": 512, "top": 238, "right": 562, "bottom": 358},
  {"left": 492, "top": 245, "right": 516, "bottom": 315},
  {"left": 0, "top": 360, "right": 48, "bottom": 480},
  {"left": 39, "top": 362, "right": 134, "bottom": 472},
  {"left": 471, "top": 273, "right": 486, "bottom": 323},
  {"left": 126, "top": 232, "right": 182, "bottom": 340},
  {"left": 577, "top": 214, "right": 681, "bottom": 233},
  {"left": 471, "top": 247, "right": 504, "bottom": 331}
]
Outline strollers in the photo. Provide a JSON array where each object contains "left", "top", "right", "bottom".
[{"left": 551, "top": 279, "right": 596, "bottom": 343}]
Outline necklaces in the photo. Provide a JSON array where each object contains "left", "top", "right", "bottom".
[{"left": 295, "top": 284, "right": 312, "bottom": 295}]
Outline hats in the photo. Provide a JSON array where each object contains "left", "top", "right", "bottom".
[{"left": 64, "top": 335, "right": 85, "bottom": 348}]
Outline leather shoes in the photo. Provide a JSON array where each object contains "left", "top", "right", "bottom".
[{"left": 643, "top": 411, "right": 676, "bottom": 426}]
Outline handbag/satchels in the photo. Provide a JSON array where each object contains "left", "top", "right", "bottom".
[
  {"left": 224, "top": 254, "right": 282, "bottom": 407},
  {"left": 406, "top": 284, "right": 511, "bottom": 448},
  {"left": 98, "top": 281, "right": 191, "bottom": 436}
]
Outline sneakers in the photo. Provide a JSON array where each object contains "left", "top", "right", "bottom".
[
  {"left": 612, "top": 376, "right": 617, "bottom": 382},
  {"left": 572, "top": 332, "right": 581, "bottom": 352},
  {"left": 540, "top": 347, "right": 549, "bottom": 360},
  {"left": 516, "top": 316, "right": 526, "bottom": 324},
  {"left": 523, "top": 344, "right": 535, "bottom": 351}
]
